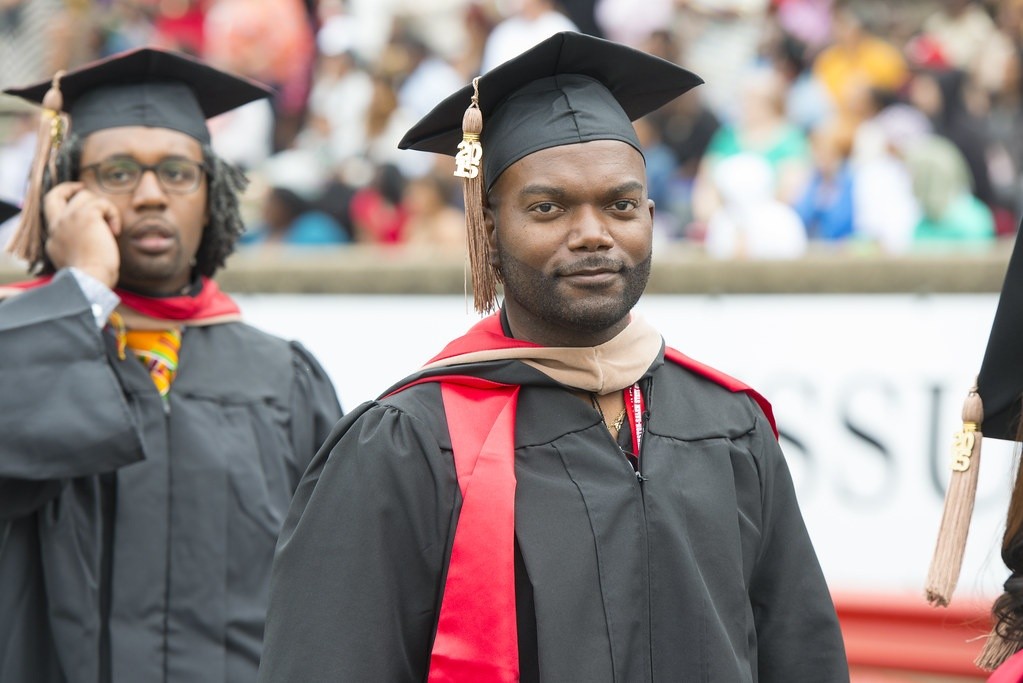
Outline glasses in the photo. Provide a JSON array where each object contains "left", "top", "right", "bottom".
[{"left": 74, "top": 155, "right": 213, "bottom": 194}]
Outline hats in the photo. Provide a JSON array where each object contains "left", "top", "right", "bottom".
[
  {"left": 1, "top": 48, "right": 276, "bottom": 263},
  {"left": 925, "top": 217, "right": 1023, "bottom": 605},
  {"left": 397, "top": 31, "right": 706, "bottom": 313}
]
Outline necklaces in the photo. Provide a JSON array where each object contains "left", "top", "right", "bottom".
[{"left": 607, "top": 408, "right": 626, "bottom": 431}]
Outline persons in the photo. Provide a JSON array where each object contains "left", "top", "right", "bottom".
[
  {"left": 255, "top": 30, "right": 853, "bottom": 683},
  {"left": 925, "top": 217, "right": 1023, "bottom": 682},
  {"left": 0, "top": 0, "right": 1023, "bottom": 242},
  {"left": 0, "top": 44, "right": 345, "bottom": 683}
]
